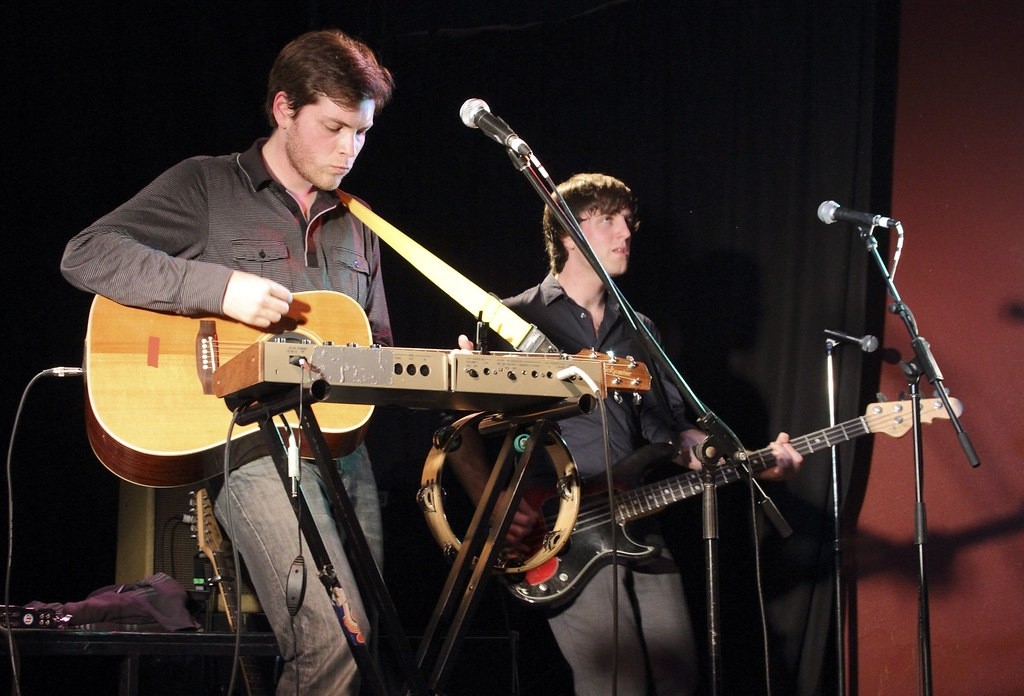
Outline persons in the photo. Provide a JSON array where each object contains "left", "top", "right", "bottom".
[
  {"left": 448, "top": 173, "right": 803, "bottom": 696},
  {"left": 61, "top": 30, "right": 479, "bottom": 696}
]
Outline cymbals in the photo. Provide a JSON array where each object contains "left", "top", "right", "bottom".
[
  {"left": 535, "top": 416, "right": 565, "bottom": 449},
  {"left": 498, "top": 556, "right": 527, "bottom": 587},
  {"left": 441, "top": 535, "right": 476, "bottom": 565},
  {"left": 553, "top": 471, "right": 587, "bottom": 500},
  {"left": 541, "top": 528, "right": 575, "bottom": 560},
  {"left": 429, "top": 423, "right": 468, "bottom": 455},
  {"left": 412, "top": 482, "right": 453, "bottom": 512}
]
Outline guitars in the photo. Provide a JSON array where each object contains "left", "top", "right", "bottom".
[
  {"left": 181, "top": 485, "right": 270, "bottom": 695},
  {"left": 73, "top": 284, "right": 655, "bottom": 496},
  {"left": 498, "top": 386, "right": 969, "bottom": 616}
]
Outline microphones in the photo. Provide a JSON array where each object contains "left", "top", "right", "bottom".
[
  {"left": 824, "top": 329, "right": 878, "bottom": 352},
  {"left": 818, "top": 201, "right": 901, "bottom": 232},
  {"left": 460, "top": 97, "right": 531, "bottom": 156}
]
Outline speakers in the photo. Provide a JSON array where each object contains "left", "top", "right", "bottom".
[{"left": 116, "top": 479, "right": 265, "bottom": 613}]
